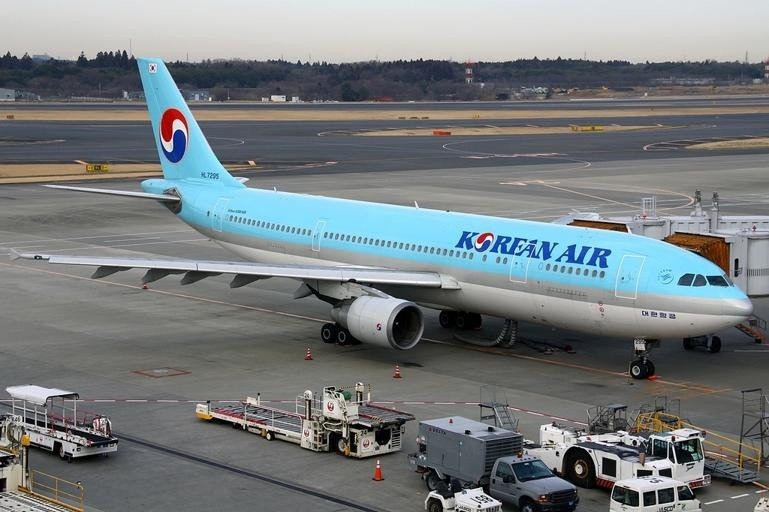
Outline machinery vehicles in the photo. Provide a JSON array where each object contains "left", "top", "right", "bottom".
[{"left": 521, "top": 403, "right": 712, "bottom": 494}]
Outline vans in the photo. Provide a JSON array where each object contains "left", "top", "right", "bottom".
[{"left": 609, "top": 474, "right": 703, "bottom": 511}]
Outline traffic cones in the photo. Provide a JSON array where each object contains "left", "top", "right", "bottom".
[
  {"left": 393, "top": 363, "right": 402, "bottom": 378},
  {"left": 304, "top": 346, "right": 314, "bottom": 361},
  {"left": 372, "top": 458, "right": 385, "bottom": 481}
]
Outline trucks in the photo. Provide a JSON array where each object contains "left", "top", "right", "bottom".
[{"left": 407, "top": 415, "right": 580, "bottom": 511}]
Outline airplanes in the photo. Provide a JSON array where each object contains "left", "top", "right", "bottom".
[{"left": 8, "top": 55, "right": 754, "bottom": 382}]
